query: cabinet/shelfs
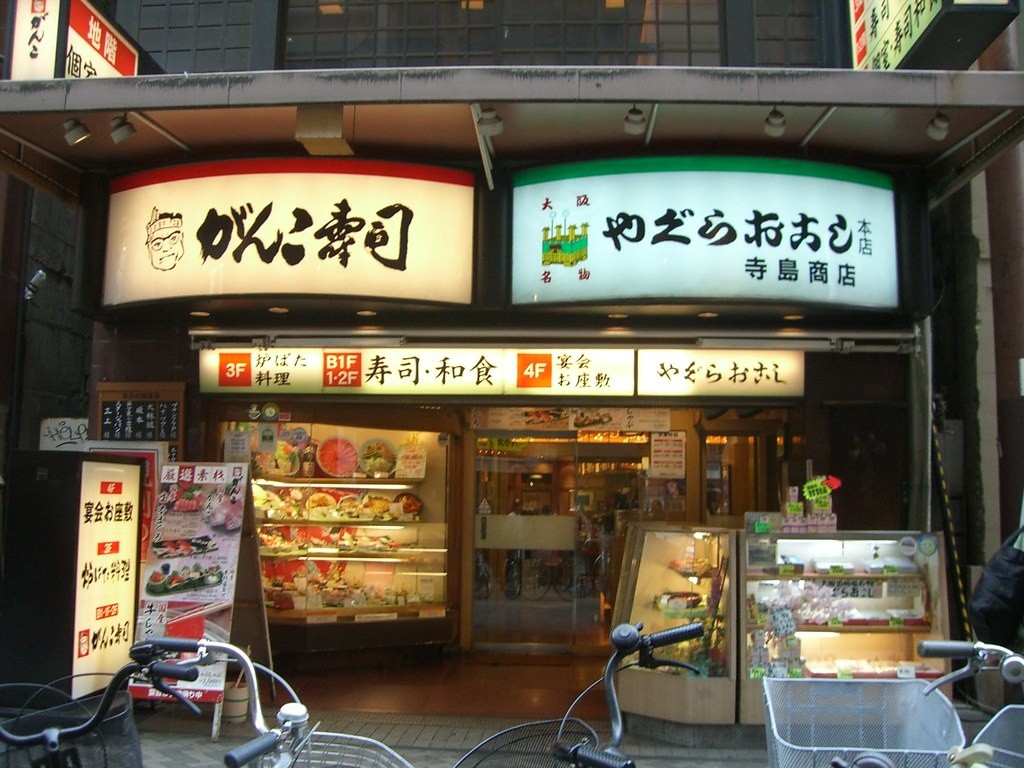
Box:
[609,524,738,727]
[737,529,954,726]
[202,400,464,654]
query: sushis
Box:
[160,538,193,557]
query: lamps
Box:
[476,101,505,137]
[924,108,951,142]
[622,104,647,135]
[110,112,136,144]
[62,119,92,147]
[762,105,787,138]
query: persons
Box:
[542,502,553,515]
[508,497,525,516]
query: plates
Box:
[357,437,398,478]
[305,492,424,522]
[316,435,360,478]
[145,571,223,597]
[285,452,300,476]
[151,535,219,557]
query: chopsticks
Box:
[165,602,231,625]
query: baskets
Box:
[762,676,966,768]
[256,731,416,768]
[453,717,599,768]
[971,704,1024,768]
[0,690,143,768]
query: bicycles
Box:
[0,642,203,768]
[761,639,1024,768]
[142,636,417,768]
[450,619,705,768]
[472,505,648,603]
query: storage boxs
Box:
[743,485,838,534]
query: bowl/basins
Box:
[136,607,229,643]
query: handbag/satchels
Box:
[967,525,1024,644]
